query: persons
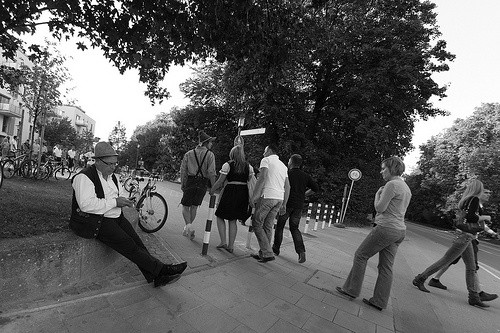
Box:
[240,166,254,225]
[412,178,500,308]
[209,146,256,252]
[70,142,188,288]
[271,154,319,263]
[2,135,163,179]
[250,145,290,263]
[180,130,217,239]
[335,157,412,310]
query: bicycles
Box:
[128,167,169,234]
[119,169,140,193]
[0,147,83,190]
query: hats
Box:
[196,134,217,148]
[91,142,119,158]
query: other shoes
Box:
[250,253,262,259]
[298,252,306,263]
[183,227,189,237]
[272,245,280,255]
[412,274,430,293]
[154,274,182,289]
[428,279,447,290]
[238,219,245,226]
[249,225,254,232]
[226,248,233,254]
[164,261,188,275]
[188,227,195,240]
[362,298,383,311]
[336,287,356,299]
[258,256,275,262]
[216,243,227,248]
[479,292,497,301]
[468,295,490,309]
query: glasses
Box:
[99,157,119,167]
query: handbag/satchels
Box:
[456,197,484,233]
[247,176,259,203]
